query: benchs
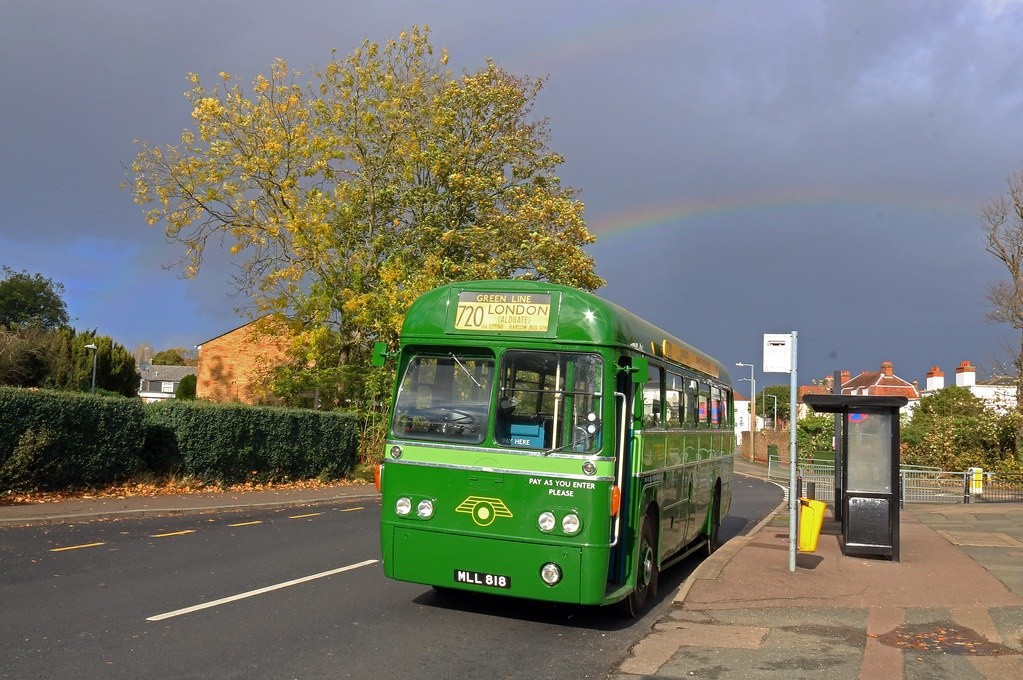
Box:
[516,411,728,429]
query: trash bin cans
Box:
[766,444,778,466]
[797,497,827,554]
[968,466,983,494]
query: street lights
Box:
[84,343,98,395]
[736,362,756,462]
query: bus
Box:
[371,280,737,620]
